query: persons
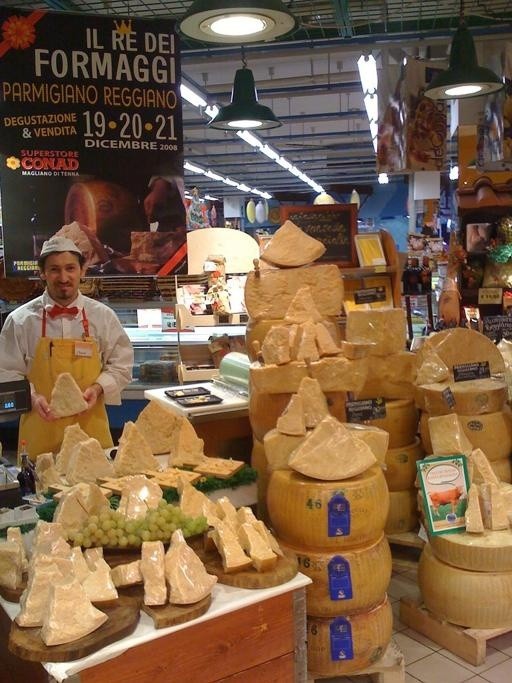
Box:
[0,234,135,462]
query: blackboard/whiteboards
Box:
[280,203,356,267]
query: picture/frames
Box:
[354,234,388,266]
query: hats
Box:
[40,237,82,257]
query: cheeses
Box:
[0,219,512,676]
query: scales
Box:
[0,380,32,412]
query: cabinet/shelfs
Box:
[255,227,402,348]
[402,257,433,340]
[438,124,512,345]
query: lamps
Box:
[356,48,389,185]
[180,71,325,202]
[207,46,282,132]
[423,0,504,101]
[177,0,300,43]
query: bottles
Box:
[17,455,36,497]
[423,314,469,335]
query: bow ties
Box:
[48,303,78,320]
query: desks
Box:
[144,379,252,458]
